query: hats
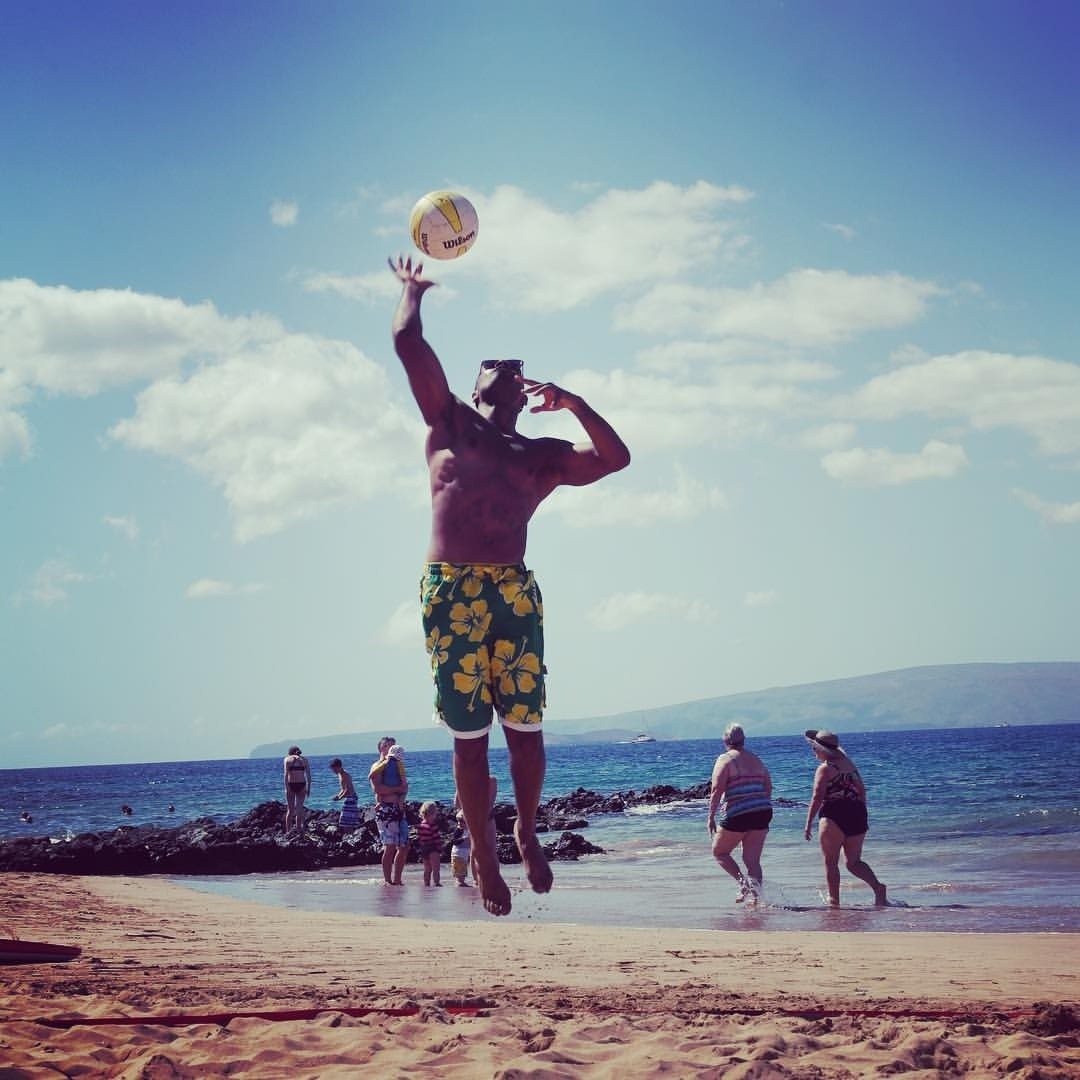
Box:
[387,745,405,761]
[804,728,847,758]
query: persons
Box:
[387,254,631,918]
[285,725,886,913]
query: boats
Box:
[630,731,655,745]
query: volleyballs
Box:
[409,188,479,260]
